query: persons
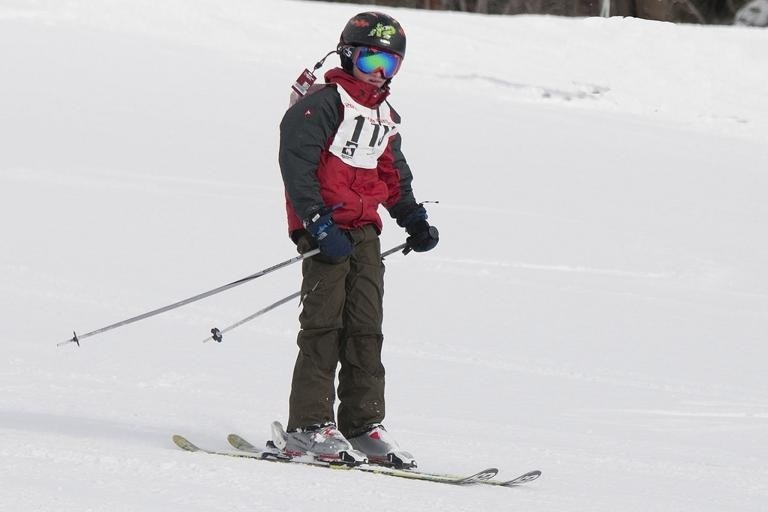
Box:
[276,12,440,467]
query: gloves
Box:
[305,213,354,257]
[405,217,439,253]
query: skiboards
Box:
[172,434,541,487]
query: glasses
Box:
[356,47,402,79]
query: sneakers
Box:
[348,423,418,469]
[286,422,367,463]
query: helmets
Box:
[338,11,406,58]
